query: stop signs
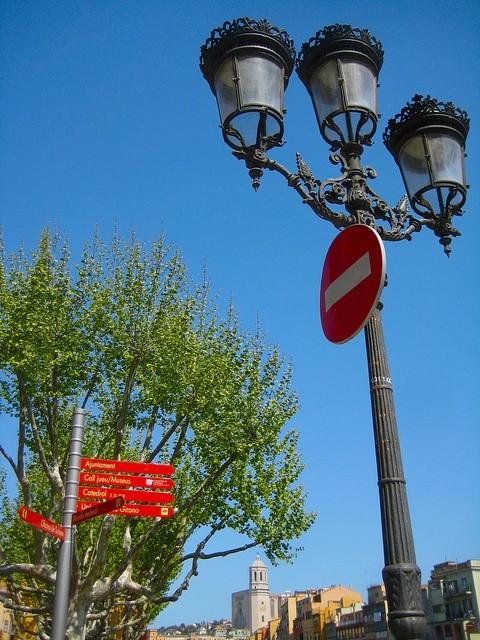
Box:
[317,223,387,345]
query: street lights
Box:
[197,8,473,636]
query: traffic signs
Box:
[78,485,173,503]
[81,472,173,490]
[72,496,122,522]
[77,501,172,518]
[19,508,64,541]
[81,458,174,475]
